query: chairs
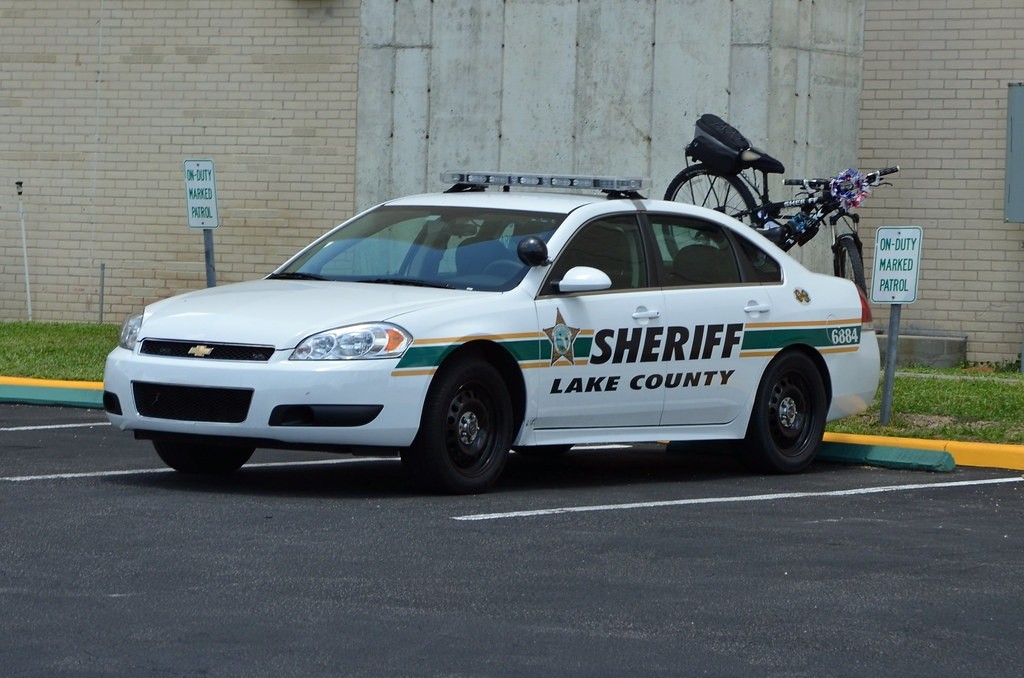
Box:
[454,237,507,277]
[672,244,727,287]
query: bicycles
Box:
[663,143,900,300]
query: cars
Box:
[102,171,880,489]
